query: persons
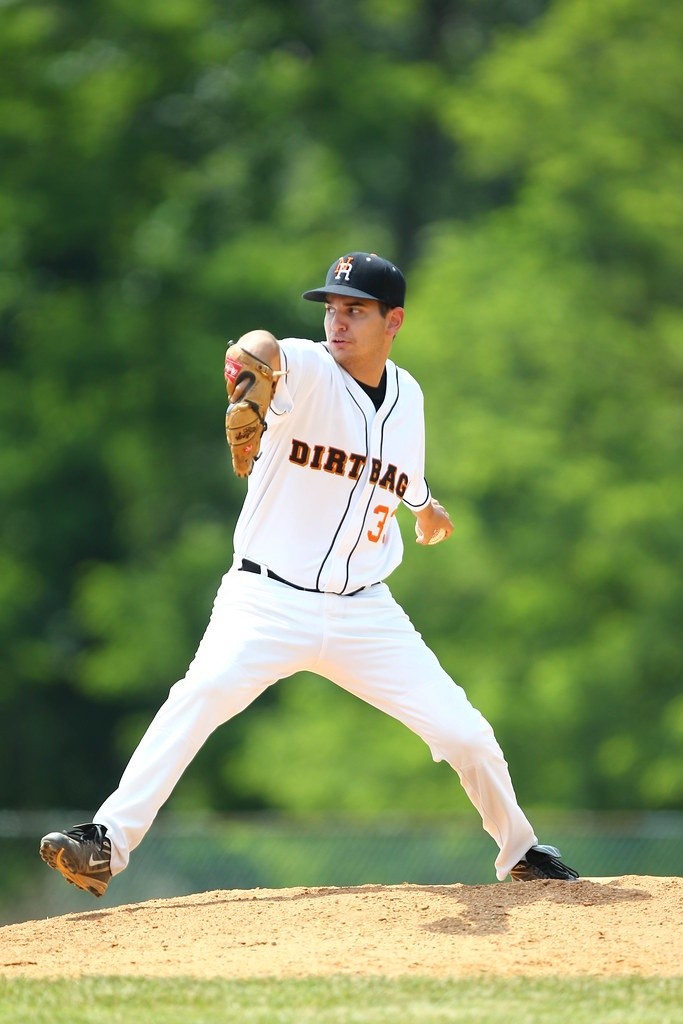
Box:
[40,251,579,897]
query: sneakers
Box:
[39,822,111,898]
[508,845,580,881]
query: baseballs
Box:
[414,518,447,546]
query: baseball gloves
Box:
[223,341,279,480]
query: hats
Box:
[302,252,407,309]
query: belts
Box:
[238,559,380,596]
